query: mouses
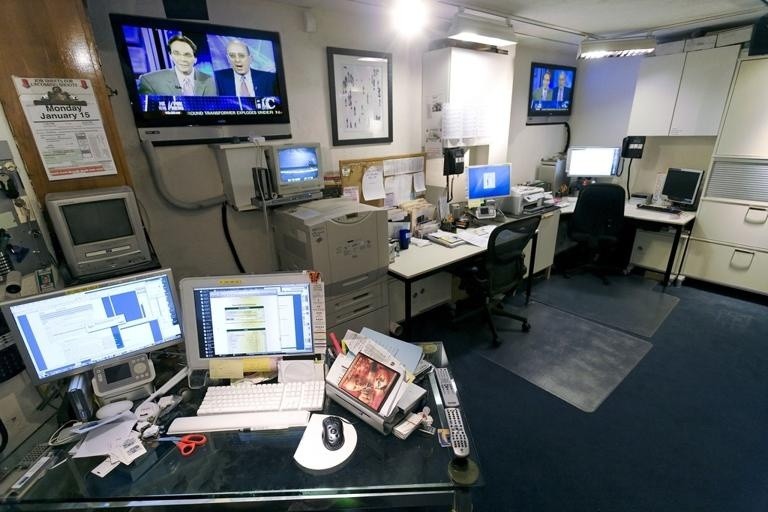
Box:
[321,416,345,451]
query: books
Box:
[325,326,449,440]
[399,198,465,248]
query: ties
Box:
[239,76,250,97]
[182,76,195,96]
[558,88,562,102]
[543,88,547,101]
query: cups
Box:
[399,229,411,250]
[441,220,456,234]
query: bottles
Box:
[554,179,587,199]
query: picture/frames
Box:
[326,46,394,146]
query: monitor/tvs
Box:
[179,272,326,370]
[46,186,151,279]
[525,62,577,127]
[465,162,512,201]
[565,145,621,178]
[265,143,326,196]
[108,12,294,142]
[661,168,705,209]
[0,267,183,388]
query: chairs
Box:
[566,183,627,286]
[457,215,542,348]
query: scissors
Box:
[154,433,207,457]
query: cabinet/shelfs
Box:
[388,273,452,324]
[625,43,741,137]
[680,53,767,296]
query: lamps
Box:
[446,12,519,49]
[575,38,656,62]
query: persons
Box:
[552,74,571,100]
[533,72,553,100]
[138,36,218,96]
[214,41,277,97]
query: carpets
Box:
[522,278,681,340]
[469,300,652,414]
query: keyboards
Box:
[637,202,682,214]
[197,380,326,418]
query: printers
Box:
[496,185,562,216]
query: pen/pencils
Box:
[443,213,454,223]
[327,332,347,358]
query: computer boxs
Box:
[539,159,570,198]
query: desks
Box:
[0,340,484,512]
[390,197,698,319]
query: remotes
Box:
[444,406,470,458]
[435,367,460,409]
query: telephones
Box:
[443,148,464,175]
[621,136,645,158]
